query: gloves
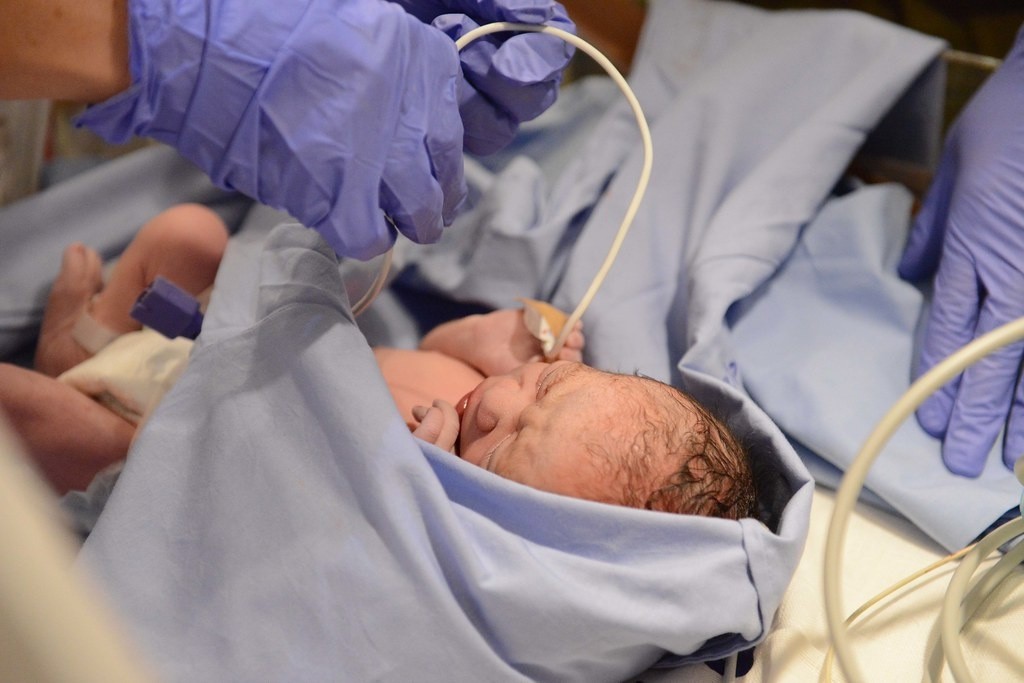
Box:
[897,26,1024,476]
[72,0,577,262]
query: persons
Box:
[0,203,759,521]
[2,4,576,261]
[896,28,1022,478]
